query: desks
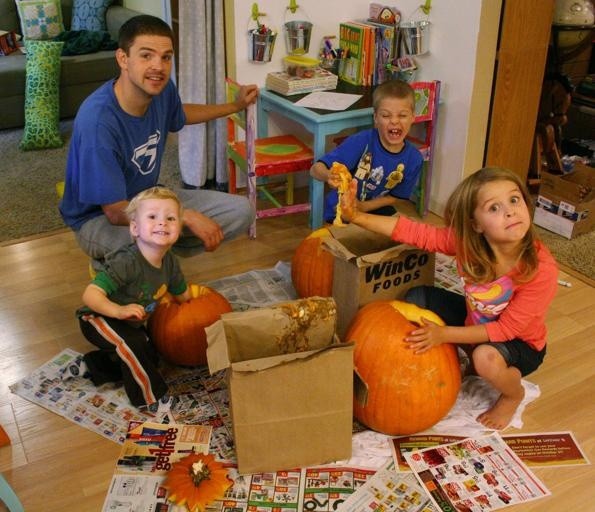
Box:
[256,85,384,234]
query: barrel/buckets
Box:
[245,12,278,64]
[400,5,431,57]
[282,6,313,55]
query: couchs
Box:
[0,0,148,130]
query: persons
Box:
[311,78,425,224]
[60,186,190,417]
[342,166,559,430]
[61,14,258,282]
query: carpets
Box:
[1,118,193,246]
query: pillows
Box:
[14,1,66,150]
[70,1,110,34]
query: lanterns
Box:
[292,224,341,298]
[345,300,463,436]
[149,284,233,364]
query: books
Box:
[265,18,396,97]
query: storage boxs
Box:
[534,161,595,239]
[321,219,438,341]
[205,293,361,473]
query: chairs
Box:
[406,81,440,219]
[222,80,314,238]
